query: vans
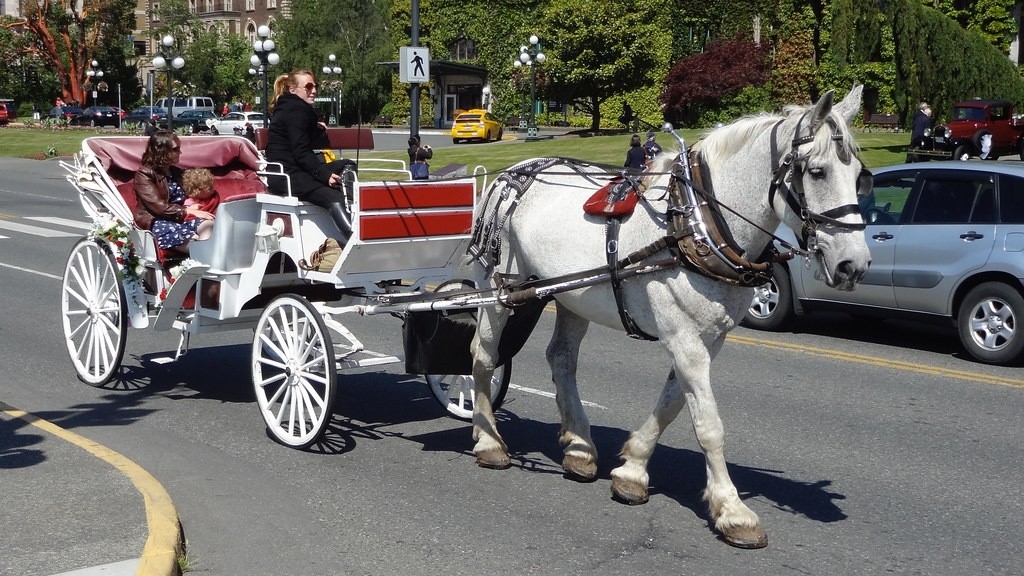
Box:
[155,97,214,119]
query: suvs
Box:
[124,106,173,131]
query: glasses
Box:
[173,147,180,152]
[296,83,317,91]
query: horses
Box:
[460,83,875,552]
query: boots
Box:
[337,164,358,197]
[326,199,353,236]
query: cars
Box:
[907,99,1024,161]
[744,161,1024,363]
[40,106,81,124]
[451,109,503,144]
[111,106,127,121]
[0,103,16,121]
[208,111,271,135]
[172,109,218,135]
[79,106,119,127]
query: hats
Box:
[645,132,656,140]
[245,122,253,126]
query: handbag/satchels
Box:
[410,147,429,179]
[299,238,341,273]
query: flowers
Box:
[89,223,186,318]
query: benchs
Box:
[862,115,899,133]
[116,178,269,264]
[255,125,412,183]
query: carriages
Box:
[59,82,873,552]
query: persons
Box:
[221,102,255,144]
[624,134,647,169]
[642,133,662,160]
[182,168,220,222]
[905,99,932,164]
[144,119,159,136]
[132,129,215,253]
[265,68,360,236]
[406,135,433,180]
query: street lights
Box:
[153,34,186,132]
[248,25,281,128]
[513,36,546,138]
[86,60,104,127]
[323,54,342,126]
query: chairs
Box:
[913,188,936,223]
